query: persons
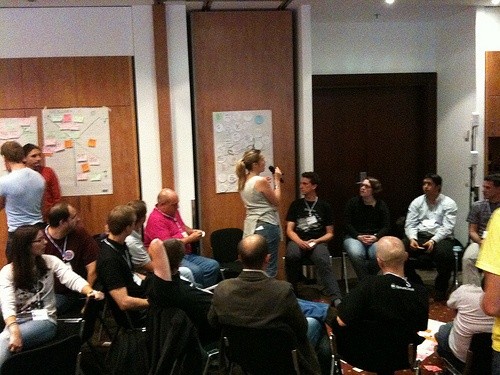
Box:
[285,172,344,307]
[207,235,338,375]
[331,236,429,347]
[343,177,391,281]
[0,141,49,264]
[237,149,285,279]
[434,173,500,375]
[0,143,61,223]
[0,187,225,369]
[400,173,457,301]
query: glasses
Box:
[32,235,46,243]
[360,183,371,188]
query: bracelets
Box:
[274,185,280,190]
[88,290,98,293]
[430,240,434,245]
[7,321,18,328]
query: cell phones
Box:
[309,241,316,247]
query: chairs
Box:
[0,228,491,375]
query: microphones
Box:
[269,166,284,183]
